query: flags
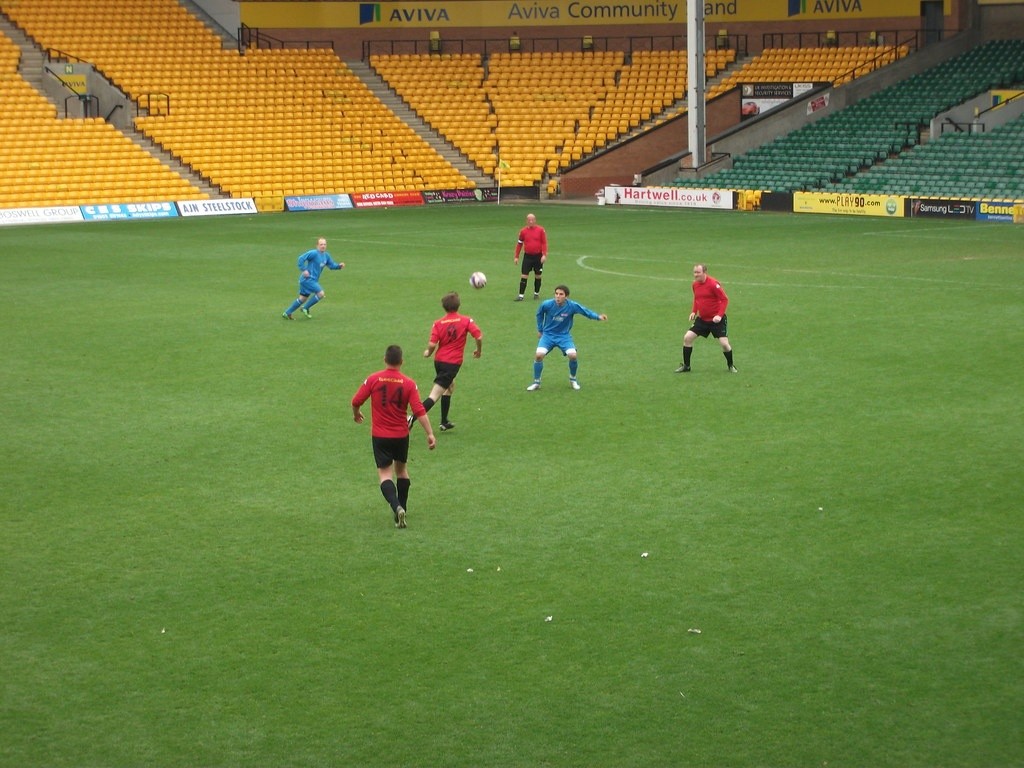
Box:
[501,160,510,167]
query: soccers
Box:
[470,272,487,289]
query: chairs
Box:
[0,1,1024,207]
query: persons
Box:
[351,345,436,528]
[408,292,482,431]
[514,214,546,301]
[282,238,344,321]
[675,264,737,373]
[527,285,607,390]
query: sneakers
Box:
[675,363,691,372]
[282,311,295,320]
[439,422,455,431]
[300,306,312,319]
[406,415,413,430]
[527,379,541,391]
[396,505,407,528]
[727,365,737,373]
[570,381,580,390]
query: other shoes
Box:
[514,296,524,301]
[534,294,539,299]
